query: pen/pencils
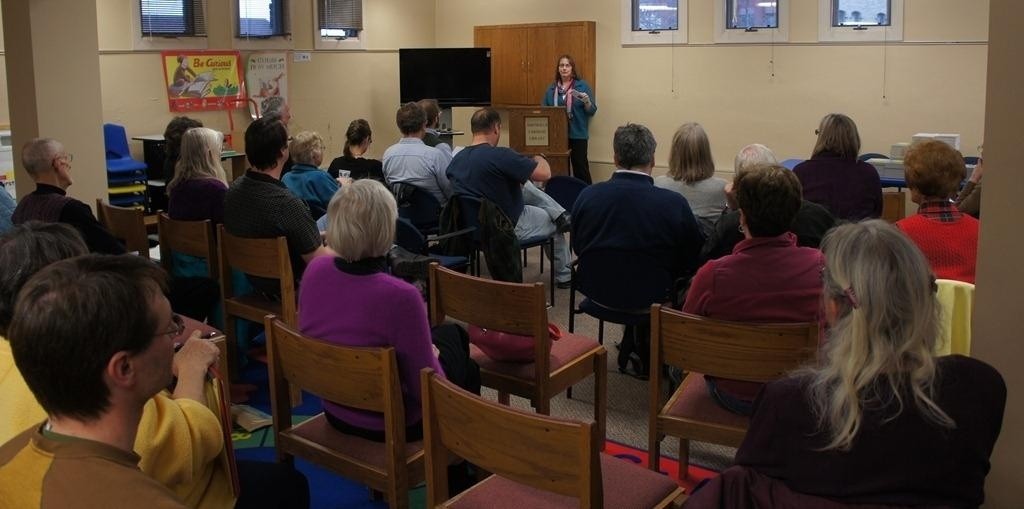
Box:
[174,331,216,353]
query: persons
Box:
[685,168,830,347]
[684,219,1008,509]
[954,138,985,218]
[539,53,595,182]
[654,121,730,224]
[172,56,195,84]
[795,111,883,226]
[1,138,310,509]
[701,143,834,264]
[569,124,703,381]
[167,97,576,289]
[300,179,481,498]
[893,140,979,284]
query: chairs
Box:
[643,301,821,473]
[262,314,457,508]
[169,311,243,500]
[427,263,612,450]
[214,223,302,385]
[441,192,557,308]
[157,209,218,332]
[100,122,150,200]
[92,200,157,264]
[564,257,648,398]
[383,181,440,253]
[413,364,683,507]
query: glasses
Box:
[52,153,73,165]
[152,313,184,338]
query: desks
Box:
[778,156,982,197]
[134,135,249,190]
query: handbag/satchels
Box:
[470,323,560,360]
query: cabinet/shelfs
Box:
[474,21,596,108]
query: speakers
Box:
[399,47,492,107]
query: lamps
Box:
[320,27,359,41]
